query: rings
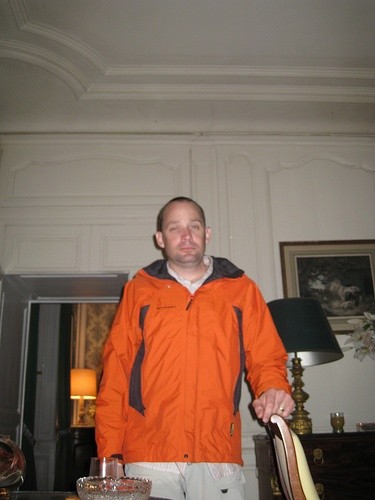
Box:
[278,407,283,412]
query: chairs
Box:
[269,414,321,500]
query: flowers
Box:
[343,312,375,362]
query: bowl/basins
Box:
[76,476,153,500]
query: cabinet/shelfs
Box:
[255,430,375,500]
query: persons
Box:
[95,197,295,500]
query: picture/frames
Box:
[279,239,375,333]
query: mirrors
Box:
[17,299,120,492]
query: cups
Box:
[330,413,344,433]
[89,456,125,477]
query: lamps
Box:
[266,297,344,434]
[70,368,97,425]
